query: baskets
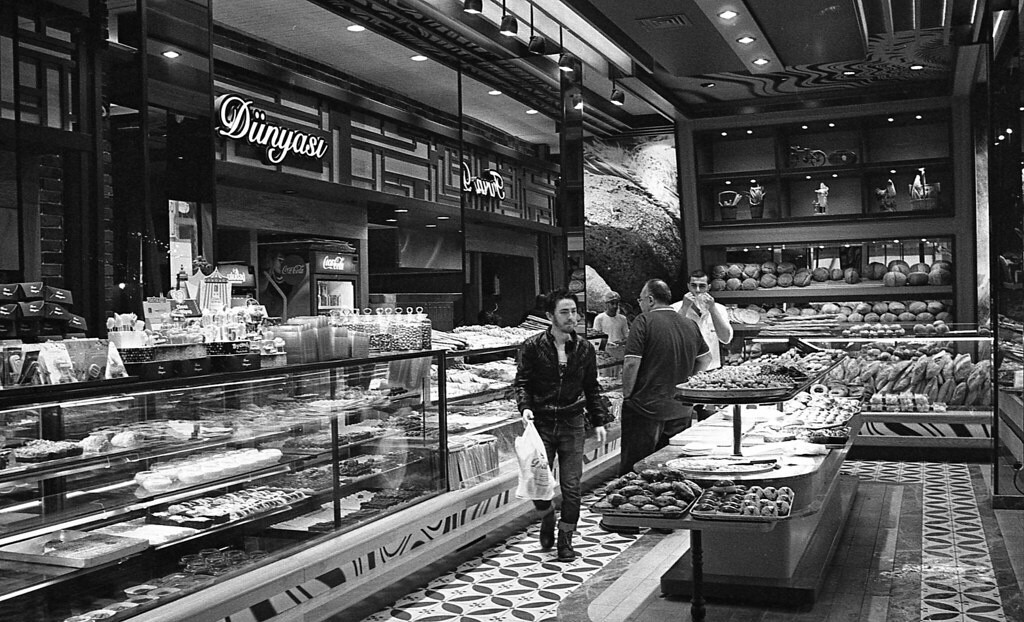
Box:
[156,343,212,359]
[211,341,250,355]
[117,341,157,362]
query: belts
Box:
[532,399,586,414]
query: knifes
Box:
[728,460,777,466]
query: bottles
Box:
[328,307,432,352]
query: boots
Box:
[540,510,556,548]
[557,530,575,562]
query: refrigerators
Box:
[214,262,255,307]
[259,251,361,318]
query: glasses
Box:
[637,296,649,303]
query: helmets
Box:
[605,291,621,304]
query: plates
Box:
[665,456,775,473]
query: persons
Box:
[514,290,607,562]
[477,270,734,423]
[599,278,712,535]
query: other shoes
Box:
[651,527,673,534]
[599,520,639,533]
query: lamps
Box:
[500,15,518,36]
[570,94,584,110]
[463,0,483,14]
[529,36,545,55]
[610,81,624,105]
[558,52,574,72]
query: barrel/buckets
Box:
[718,191,737,220]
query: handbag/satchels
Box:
[515,420,555,500]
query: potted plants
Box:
[719,191,742,221]
[746,185,766,219]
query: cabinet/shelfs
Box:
[675,99,976,366]
